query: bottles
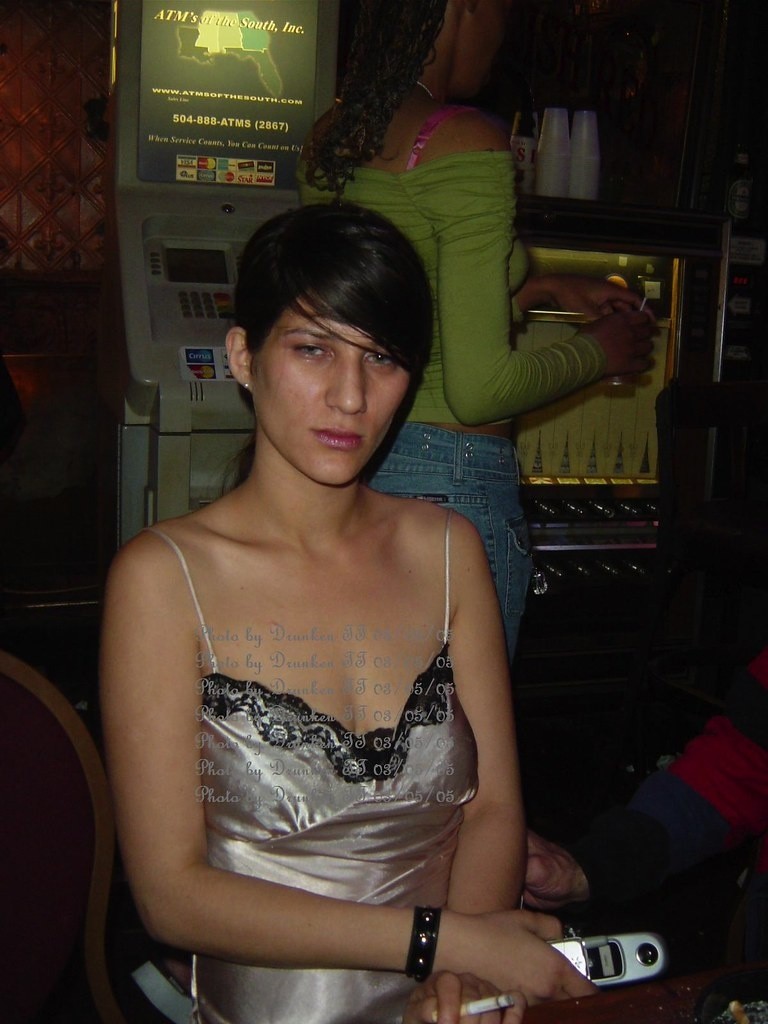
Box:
[720,126,754,234]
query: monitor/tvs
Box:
[137,0,320,191]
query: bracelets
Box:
[404,905,441,983]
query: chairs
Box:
[0,649,126,1024]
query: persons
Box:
[98,206,600,1023]
[520,641,768,1023]
[317,0,657,676]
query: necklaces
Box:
[414,77,436,100]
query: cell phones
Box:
[544,933,665,984]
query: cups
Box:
[602,375,635,386]
[510,111,597,201]
